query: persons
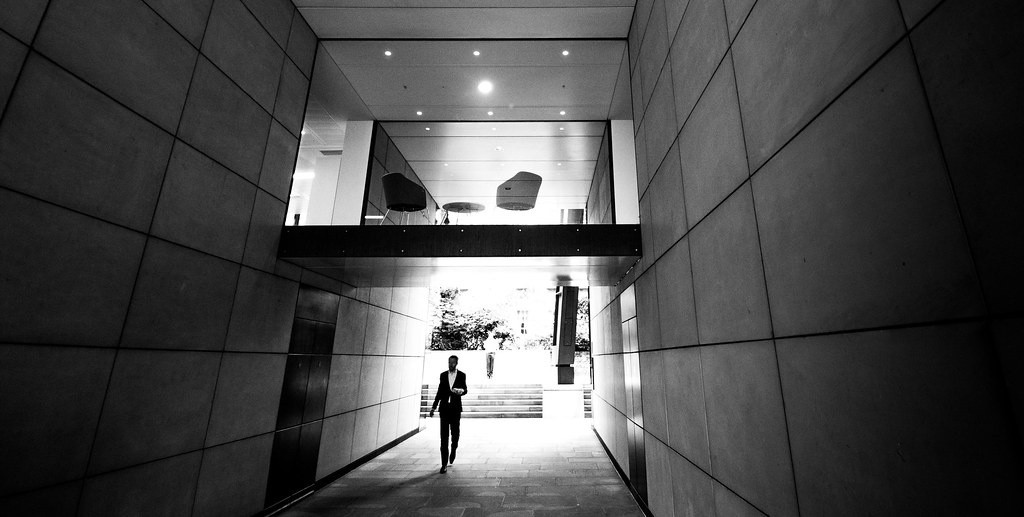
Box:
[483,333,499,379]
[430,355,468,474]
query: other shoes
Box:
[450,449,455,464]
[440,464,448,474]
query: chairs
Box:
[496,171,542,210]
[377,173,435,224]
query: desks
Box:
[438,201,485,224]
